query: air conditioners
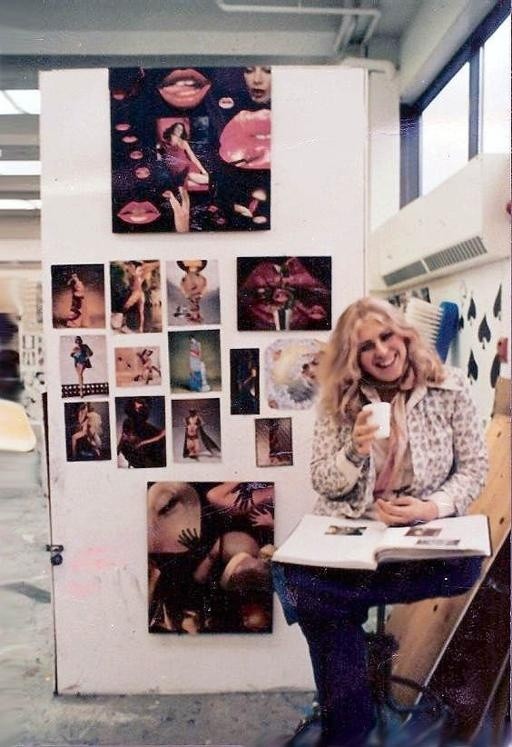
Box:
[371,153,512,293]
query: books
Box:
[271,513,494,571]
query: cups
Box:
[364,402,392,437]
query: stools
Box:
[290,575,478,746]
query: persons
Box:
[182,407,220,459]
[121,260,147,332]
[241,66,271,104]
[117,201,161,226]
[147,480,275,558]
[159,122,209,190]
[157,69,211,109]
[70,336,94,397]
[178,335,203,393]
[284,296,489,747]
